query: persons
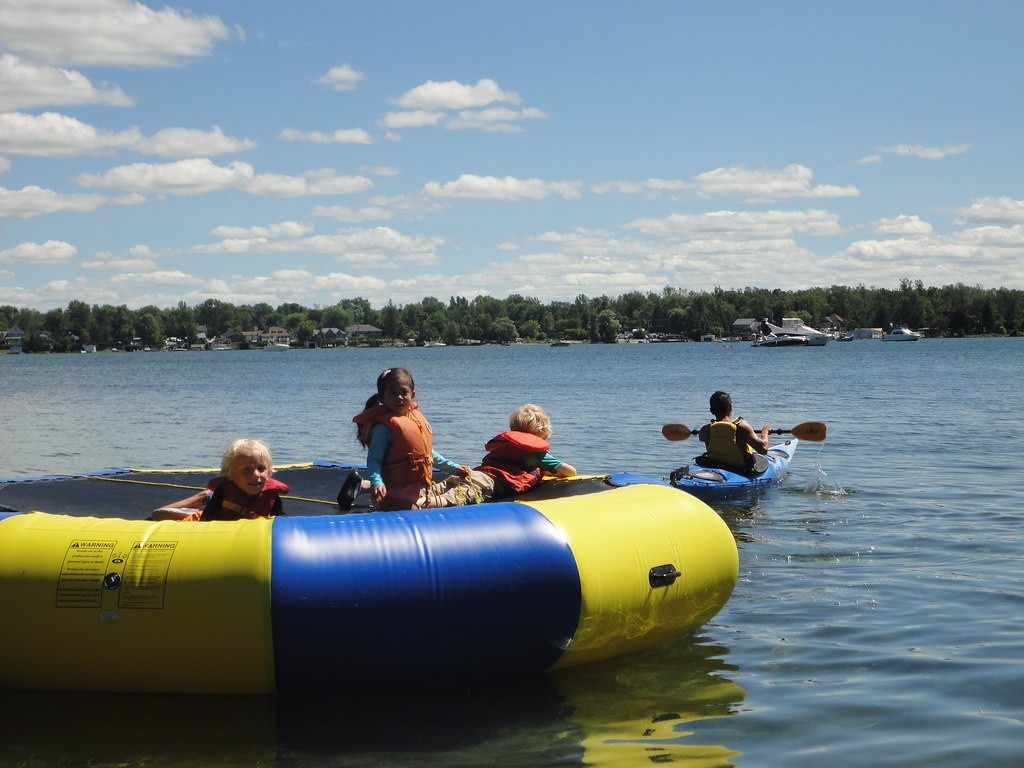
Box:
[338,404,576,510]
[700,391,772,470]
[151,438,289,521]
[352,368,472,501]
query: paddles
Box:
[661,421,828,442]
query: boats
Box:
[663,439,798,496]
[550,340,571,347]
[884,328,920,341]
[263,340,289,351]
[424,342,446,348]
[763,318,835,346]
[751,335,810,347]
[836,335,853,341]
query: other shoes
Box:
[337,470,362,510]
